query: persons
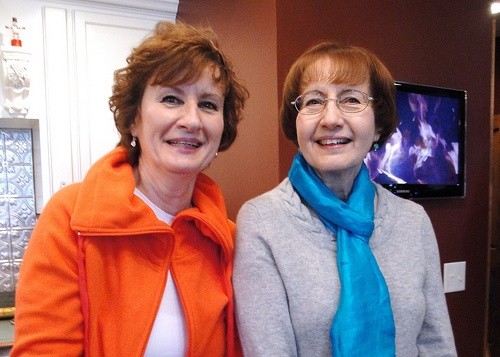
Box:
[231,41,458,357]
[8,19,250,357]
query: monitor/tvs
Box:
[363,81,467,199]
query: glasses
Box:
[291,92,376,114]
